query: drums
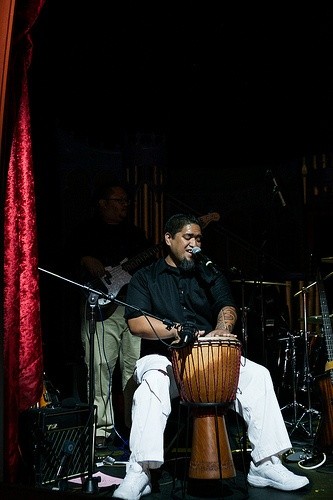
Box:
[239,304,266,343]
[274,334,306,382]
[170,336,241,479]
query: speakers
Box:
[19,404,98,485]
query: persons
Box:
[112,213,309,500]
[67,181,158,449]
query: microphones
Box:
[57,440,74,477]
[179,326,195,348]
[191,246,218,274]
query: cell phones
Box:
[280,281,321,438]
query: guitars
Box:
[88,211,221,305]
[315,274,333,448]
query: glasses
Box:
[106,197,131,204]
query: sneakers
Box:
[113,462,152,500]
[247,456,309,491]
